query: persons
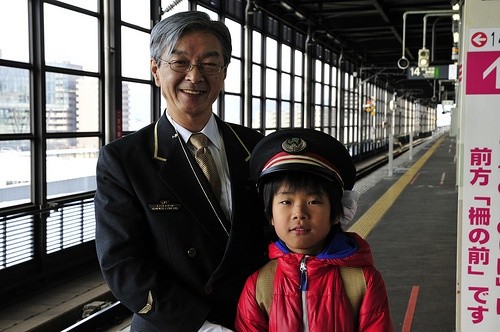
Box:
[234,127,395,332]
[96,10,359,332]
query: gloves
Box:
[338,189,360,228]
[199,320,233,332]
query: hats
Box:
[249,128,357,192]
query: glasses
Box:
[157,57,229,76]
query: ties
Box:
[189,133,222,204]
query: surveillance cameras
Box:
[398,58,409,69]
[432,97,437,102]
